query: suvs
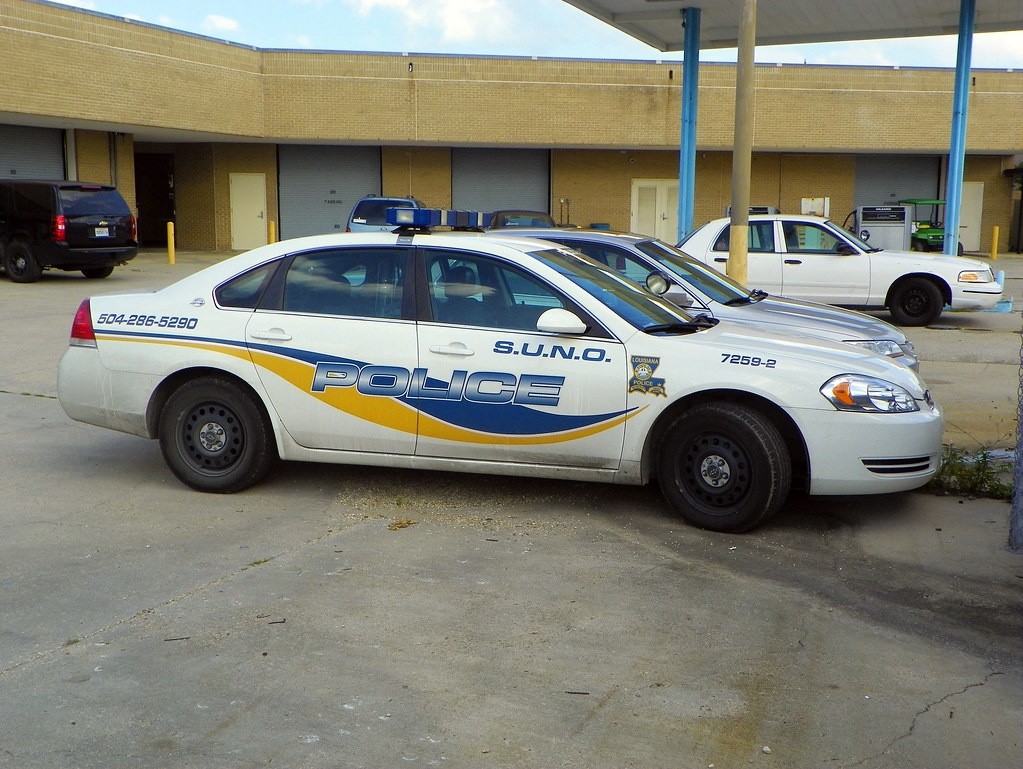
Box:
[345,194,428,259]
[0,178,139,283]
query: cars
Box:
[625,214,1002,327]
[483,210,559,231]
[53,207,941,534]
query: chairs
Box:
[441,266,482,325]
[311,275,355,316]
